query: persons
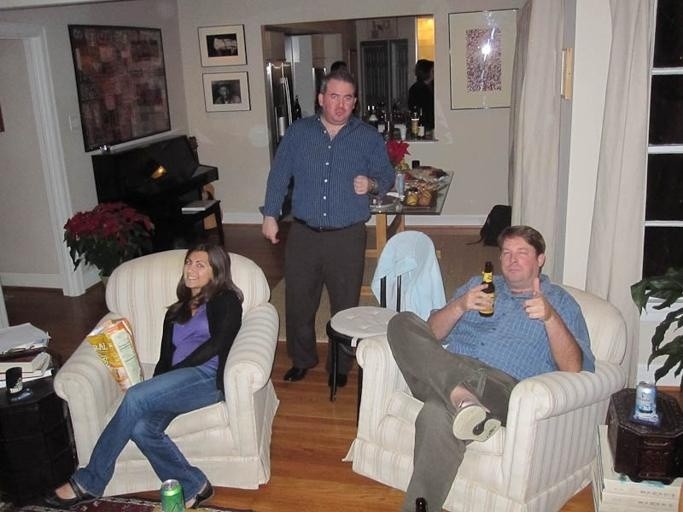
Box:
[41,242,242,509]
[387,225,595,511]
[258,70,394,387]
[330,61,347,71]
[215,83,240,103]
[409,59,435,135]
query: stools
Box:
[179,198,226,248]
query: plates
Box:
[612,388,683,439]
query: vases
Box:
[98,272,114,285]
[630,266,683,387]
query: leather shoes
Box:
[283,367,306,381]
[328,372,347,388]
[188,480,213,509]
[42,478,100,510]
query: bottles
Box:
[476,259,498,317]
[294,96,302,119]
[410,104,419,138]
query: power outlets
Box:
[69,113,81,135]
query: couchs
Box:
[341,282,628,511]
[53,249,280,500]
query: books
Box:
[180,200,218,211]
[0,352,54,389]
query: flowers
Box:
[63,201,156,276]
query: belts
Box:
[293,214,351,234]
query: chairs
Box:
[326,230,447,428]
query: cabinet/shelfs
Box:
[358,38,408,120]
[0,347,79,506]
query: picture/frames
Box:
[197,24,248,68]
[371,16,400,36]
[202,71,252,113]
[67,23,172,153]
[447,6,521,111]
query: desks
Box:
[359,168,454,306]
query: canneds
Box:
[633,381,659,416]
[158,479,185,512]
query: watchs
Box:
[370,178,378,195]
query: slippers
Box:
[450,403,503,442]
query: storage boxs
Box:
[605,389,683,485]
